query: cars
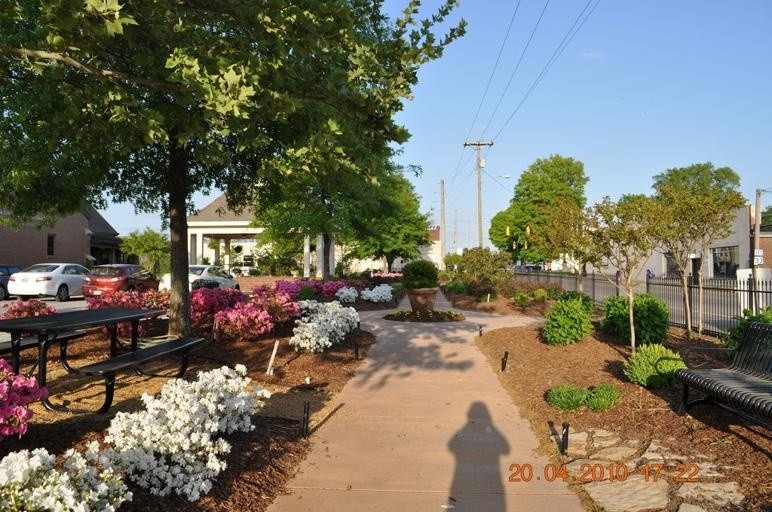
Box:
[0,263,240,301]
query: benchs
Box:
[654,318,772,451]
[75,337,206,413]
[2,330,89,384]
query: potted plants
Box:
[401,259,441,313]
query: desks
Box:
[0,306,166,414]
